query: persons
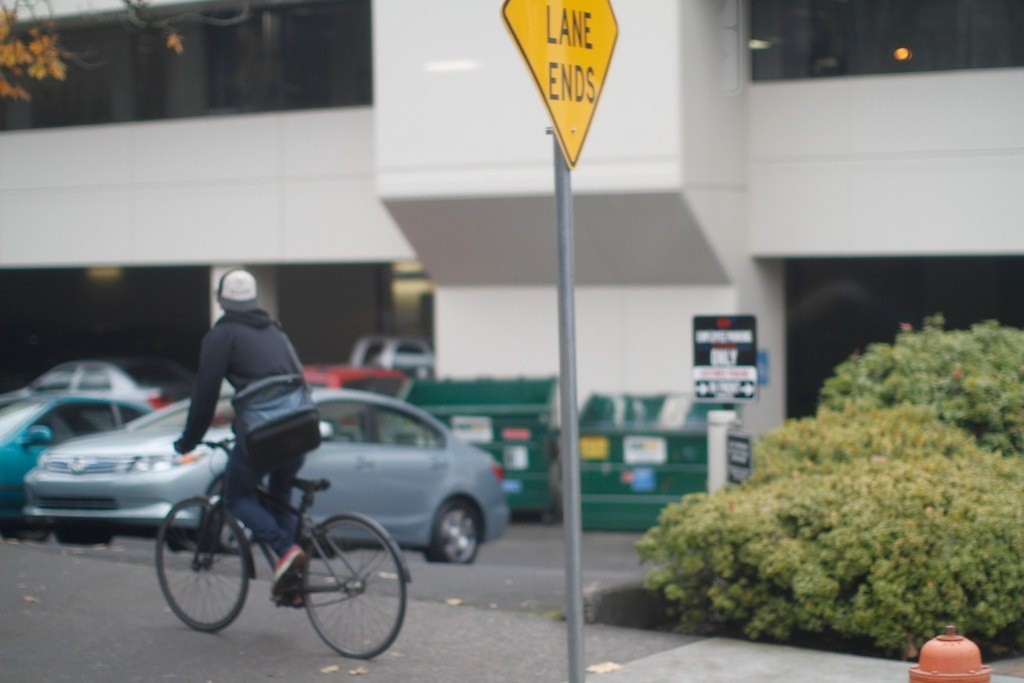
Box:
[172,269,323,610]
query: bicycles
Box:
[153,422,414,660]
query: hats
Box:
[216,268,257,313]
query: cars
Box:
[23,381,515,574]
[30,322,724,526]
[0,383,154,527]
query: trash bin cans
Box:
[394,367,561,524]
[566,387,741,534]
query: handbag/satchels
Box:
[232,374,322,466]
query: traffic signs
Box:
[727,429,754,491]
[692,313,760,404]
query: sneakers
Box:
[271,546,306,608]
